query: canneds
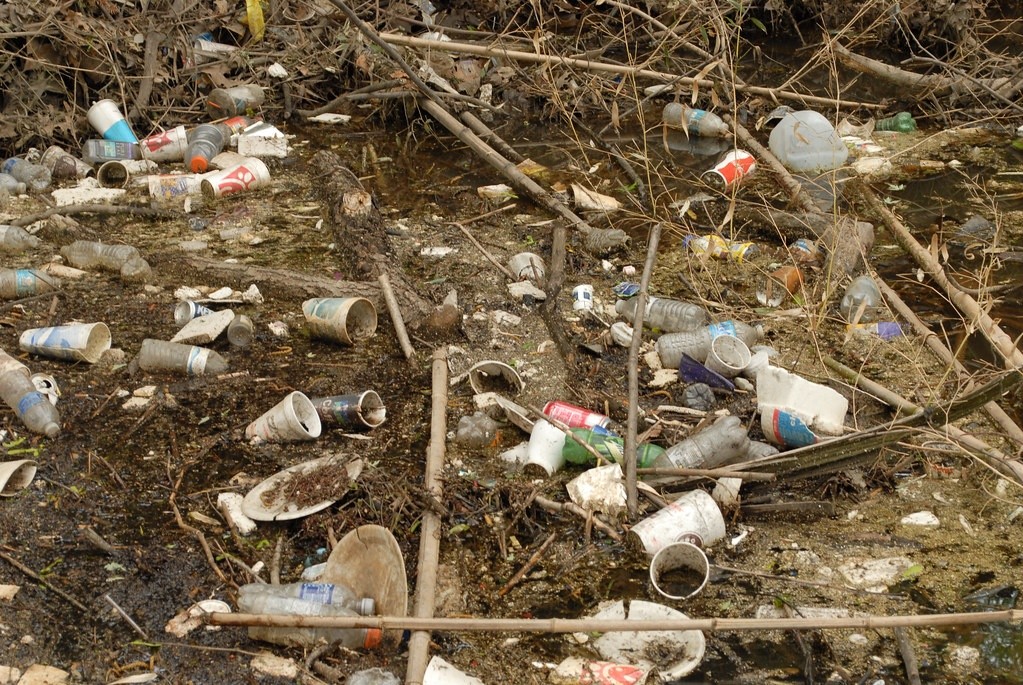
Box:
[545,401,612,431]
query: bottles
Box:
[0,39,919,650]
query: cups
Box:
[552,656,663,685]
[0,23,932,597]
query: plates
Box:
[243,453,363,524]
[576,600,706,680]
[322,526,407,648]
